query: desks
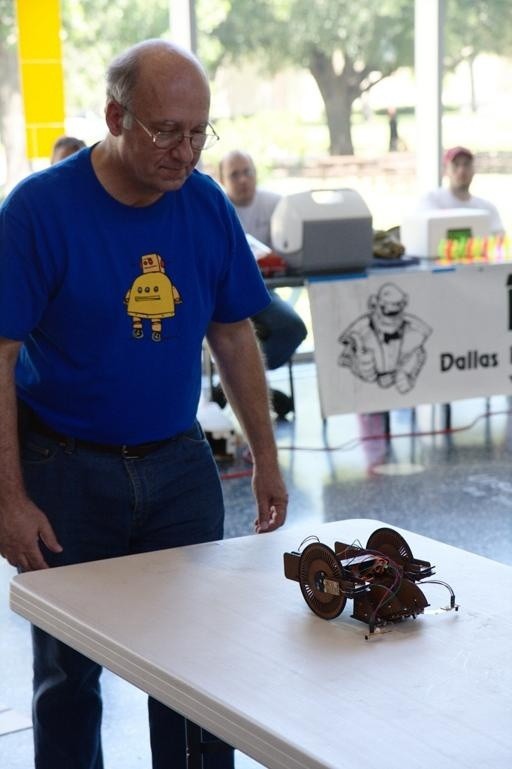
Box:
[195,252,511,464]
[7,514,512,769]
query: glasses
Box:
[123,106,221,151]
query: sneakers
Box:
[267,387,292,417]
[210,382,227,410]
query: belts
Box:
[23,404,196,461]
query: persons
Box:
[48,134,87,165]
[0,35,290,768]
[422,144,504,241]
[213,146,308,419]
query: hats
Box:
[443,145,473,166]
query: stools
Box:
[204,332,299,416]
